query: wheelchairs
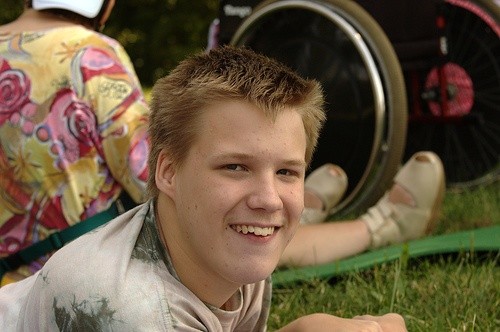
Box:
[205,1,500,220]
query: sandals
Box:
[362,150,447,251]
[296,162,348,225]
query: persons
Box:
[0,46,409,332]
[0,0,446,271]
